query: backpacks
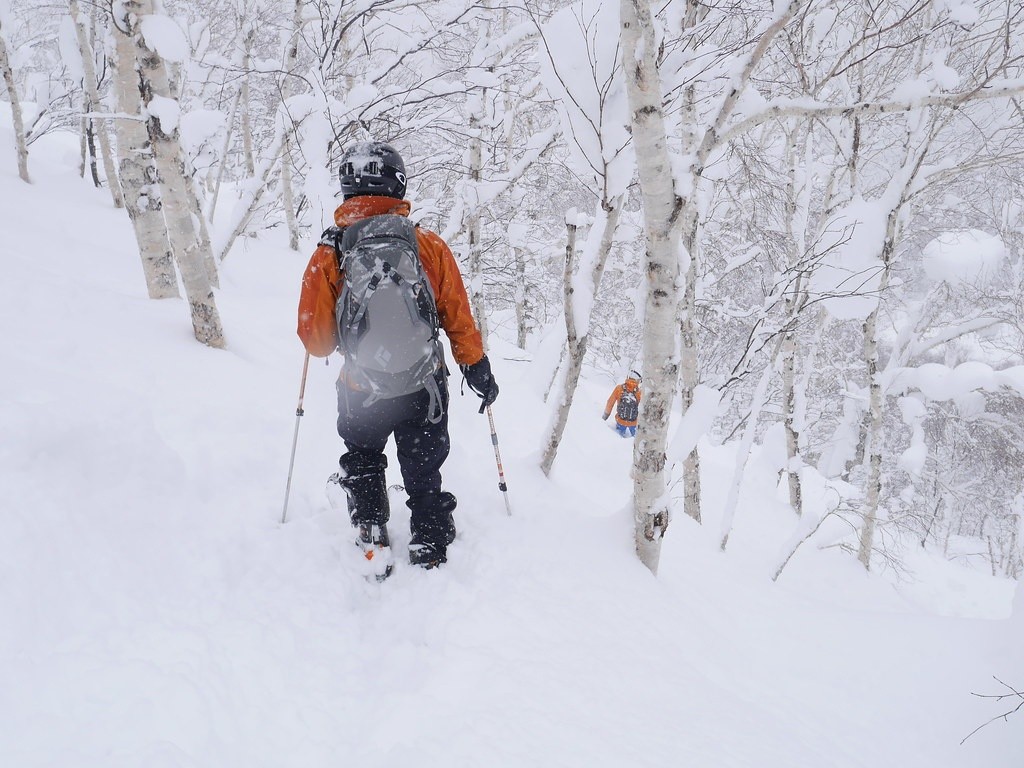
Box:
[316,213,450,423]
[616,383,640,423]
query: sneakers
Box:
[338,470,392,582]
[405,492,456,570]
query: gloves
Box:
[459,352,499,414]
[602,412,608,421]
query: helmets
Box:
[338,142,407,201]
[628,367,643,382]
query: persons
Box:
[602,370,641,464]
[297,142,498,584]
